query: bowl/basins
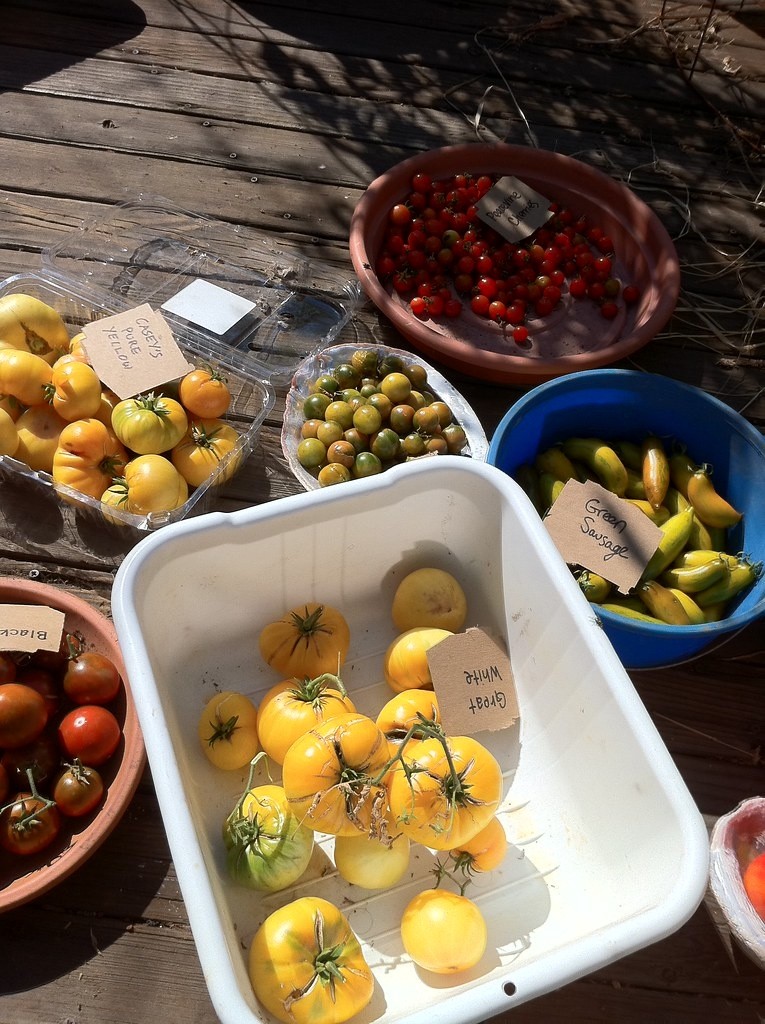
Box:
[281,343,487,492]
[0,190,376,531]
[112,455,712,1024]
[711,797,765,975]
[484,367,764,671]
[1,574,146,916]
[349,141,679,383]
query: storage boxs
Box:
[106,452,716,1023]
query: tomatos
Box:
[0,166,639,1024]
